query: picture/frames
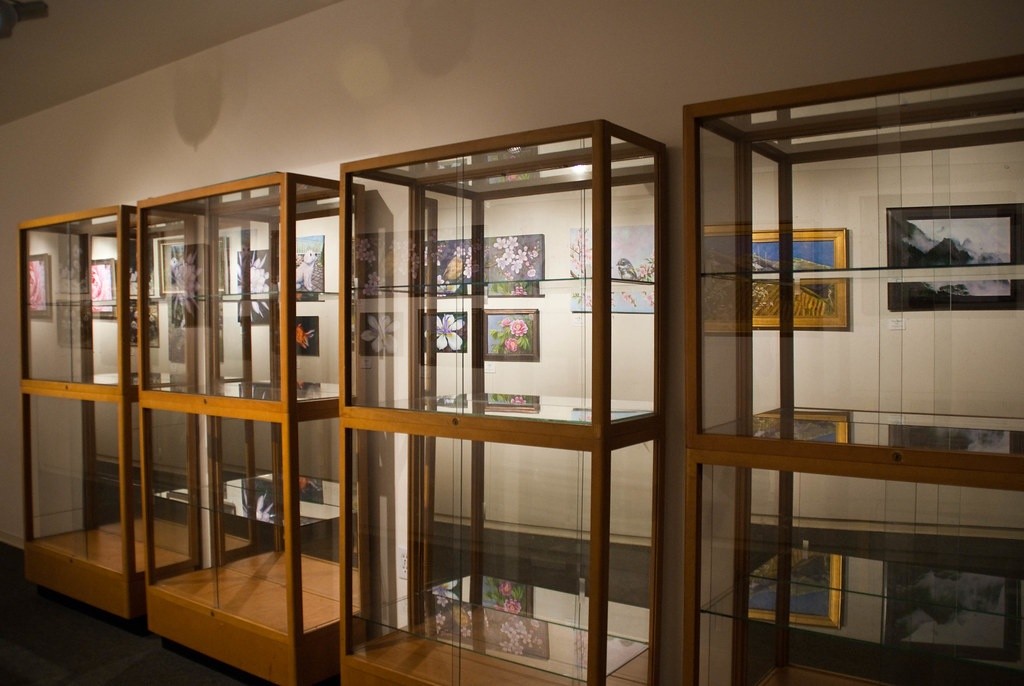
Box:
[92,259,118,319]
[28,253,53,319]
[299,477,324,505]
[752,228,849,330]
[431,594,474,647]
[483,606,550,661]
[481,308,540,363]
[296,315,321,357]
[482,576,533,620]
[436,313,466,354]
[240,476,277,524]
[746,411,850,631]
[886,202,1023,312]
[432,578,463,602]
[485,234,546,296]
[882,424,1019,662]
[158,240,231,295]
